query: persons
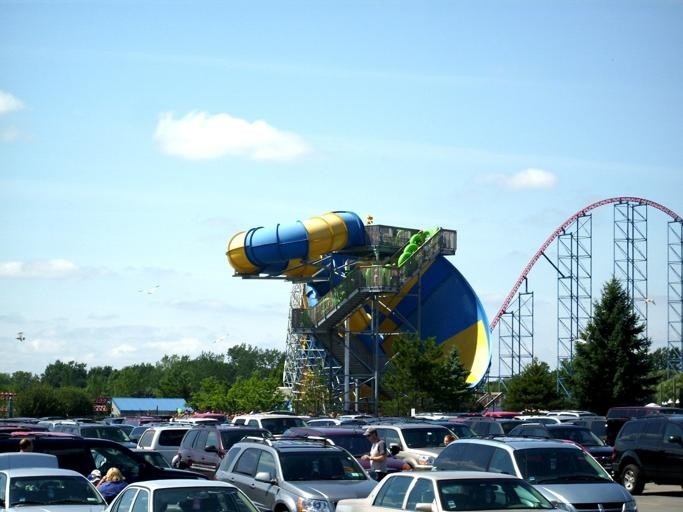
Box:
[363,429,386,480]
[97,468,127,499]
[443,437,452,446]
[20,439,34,452]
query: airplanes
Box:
[15,331,27,343]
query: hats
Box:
[91,469,102,477]
[362,427,377,436]
